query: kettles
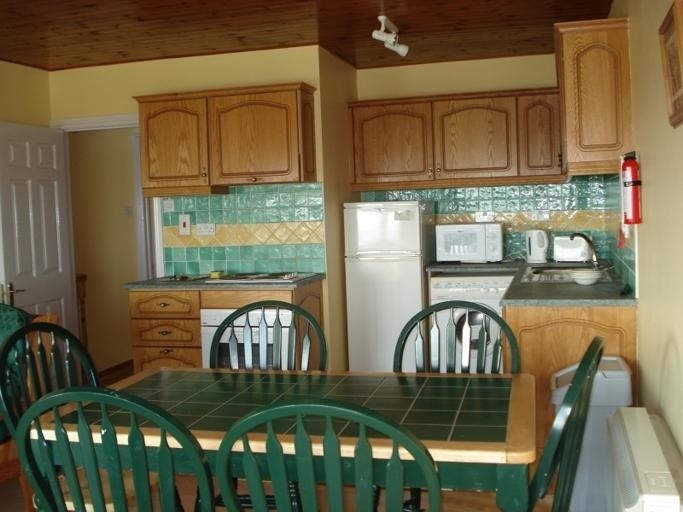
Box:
[524,229,548,264]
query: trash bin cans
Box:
[549,355,634,512]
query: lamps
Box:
[371,15,414,59]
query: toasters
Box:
[550,232,593,262]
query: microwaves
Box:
[434,223,503,263]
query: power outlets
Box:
[196,222,216,236]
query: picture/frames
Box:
[656,0,681,127]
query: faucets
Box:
[570,233,598,266]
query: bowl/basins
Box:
[570,268,601,285]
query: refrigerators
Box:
[340,199,435,374]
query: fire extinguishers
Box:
[621,150,641,224]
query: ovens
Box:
[200,308,293,371]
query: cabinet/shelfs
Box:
[126,290,200,376]
[499,302,636,497]
[551,17,633,176]
[517,87,569,183]
[74,270,90,386]
[349,89,517,189]
[131,80,316,200]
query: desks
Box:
[27,367,535,511]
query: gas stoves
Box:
[206,271,317,282]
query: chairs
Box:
[216,403,443,511]
[196,298,325,512]
[13,386,213,511]
[441,337,603,512]
[391,300,518,510]
[0,321,184,512]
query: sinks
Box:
[520,266,614,283]
